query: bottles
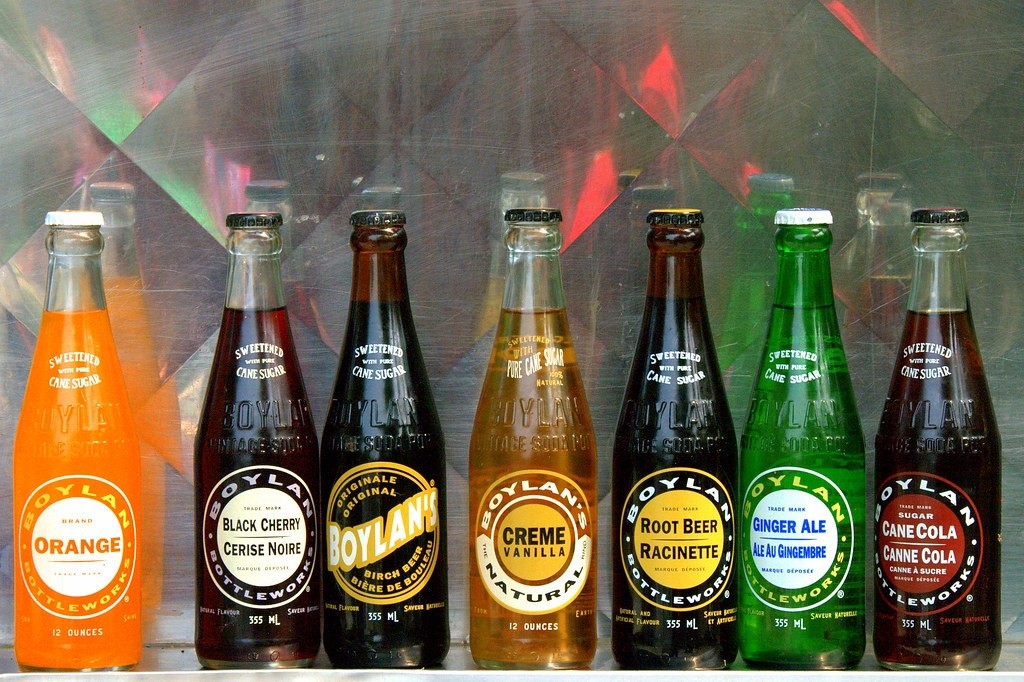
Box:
[14,210,142,671]
[322,210,450,668]
[92,182,164,620]
[483,172,549,340]
[874,209,1002,671]
[845,172,917,356]
[630,185,678,302]
[611,209,736,670]
[361,184,406,215]
[737,208,867,670]
[193,213,319,670]
[719,172,796,436]
[469,208,598,670]
[243,180,316,340]
